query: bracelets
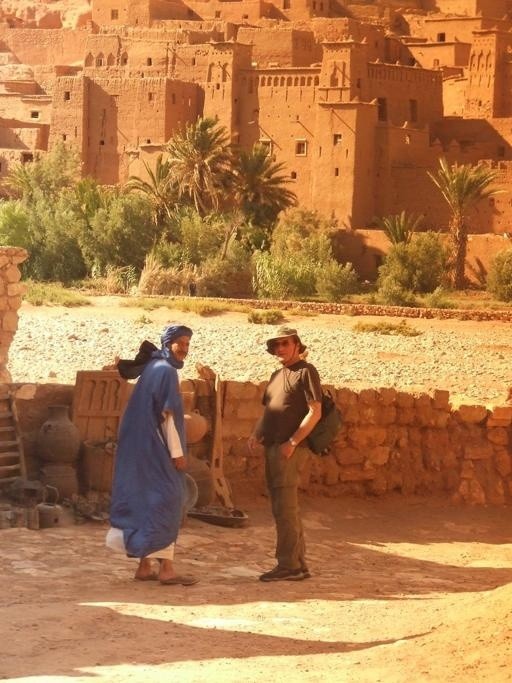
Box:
[289,436,299,450]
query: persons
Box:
[110,323,199,586]
[188,278,197,296]
[246,324,323,580]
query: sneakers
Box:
[259,565,310,582]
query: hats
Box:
[268,326,306,355]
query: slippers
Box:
[135,570,159,579]
[161,574,200,585]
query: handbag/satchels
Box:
[301,390,344,455]
